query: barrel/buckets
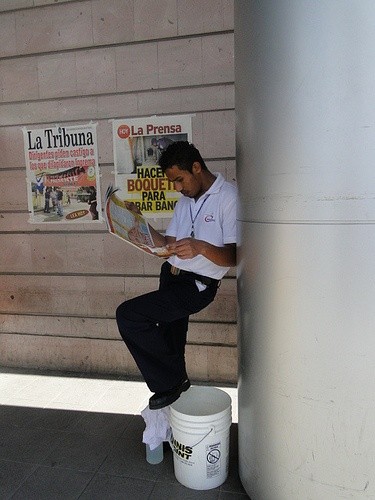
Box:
[171,385,232,490]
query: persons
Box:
[115,139,244,447]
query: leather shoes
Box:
[146,376,192,411]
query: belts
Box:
[166,265,218,288]
